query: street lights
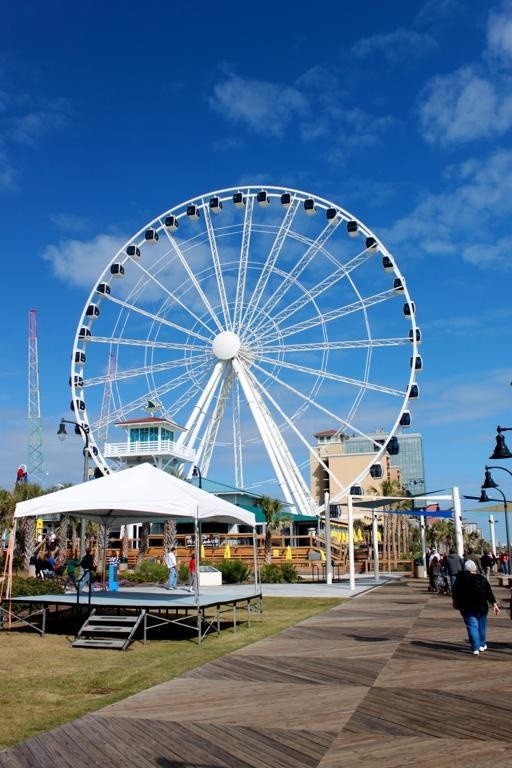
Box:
[477,422,511,577]
[55,417,92,578]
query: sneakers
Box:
[473,643,487,655]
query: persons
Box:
[30,547,99,594]
[426,545,511,656]
[110,549,120,580]
[188,553,196,593]
[187,537,218,546]
[165,547,181,590]
